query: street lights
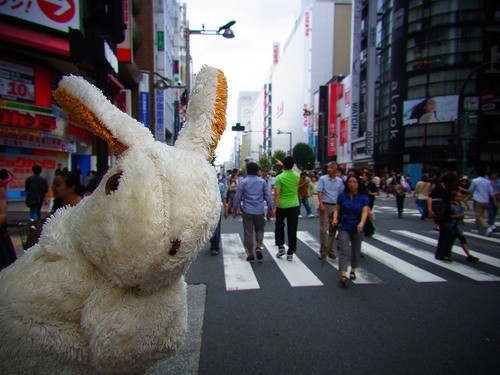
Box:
[154,27,235,113]
[277,131,292,156]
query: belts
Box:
[322,202,336,206]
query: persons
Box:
[0,162,108,270]
[409,98,439,123]
[210,156,500,283]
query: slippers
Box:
[350,272,356,279]
[340,275,347,284]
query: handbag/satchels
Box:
[401,175,410,191]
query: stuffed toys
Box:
[0,66,228,375]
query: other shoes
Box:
[246,255,254,260]
[276,247,285,257]
[433,226,439,230]
[287,254,293,261]
[471,230,479,233]
[437,256,452,262]
[399,214,403,216]
[486,228,493,237]
[328,249,335,259]
[467,255,479,261]
[212,250,219,255]
[491,224,496,229]
[421,212,426,220]
[255,247,264,260]
[360,253,364,258]
[264,213,315,222]
[318,253,327,260]
